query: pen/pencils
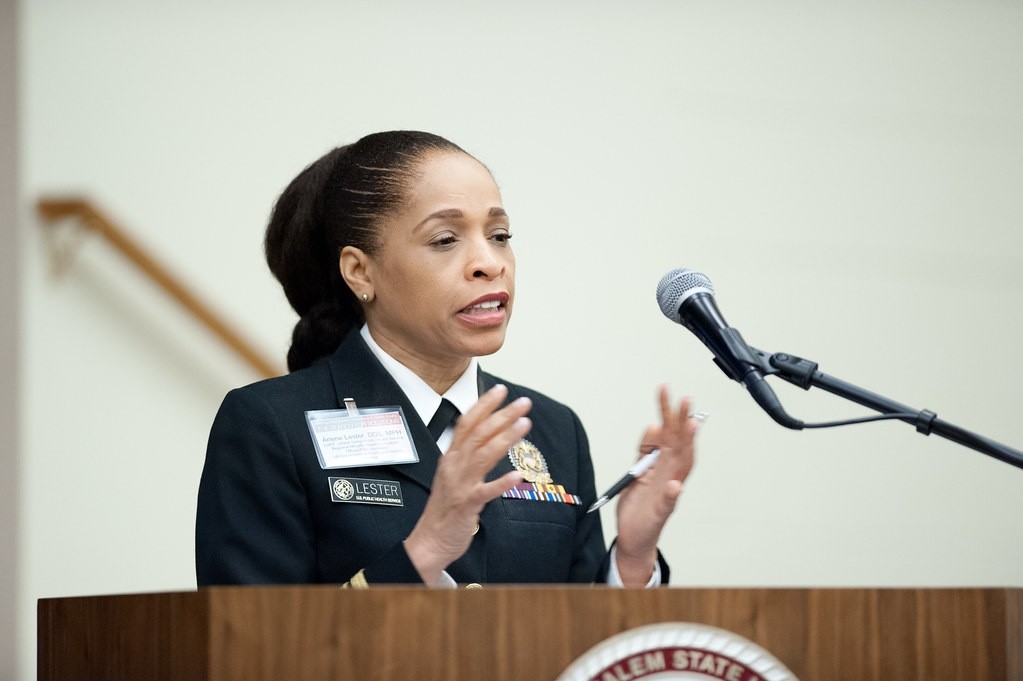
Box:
[586,413,707,513]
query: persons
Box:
[195,129,696,589]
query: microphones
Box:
[656,266,804,430]
